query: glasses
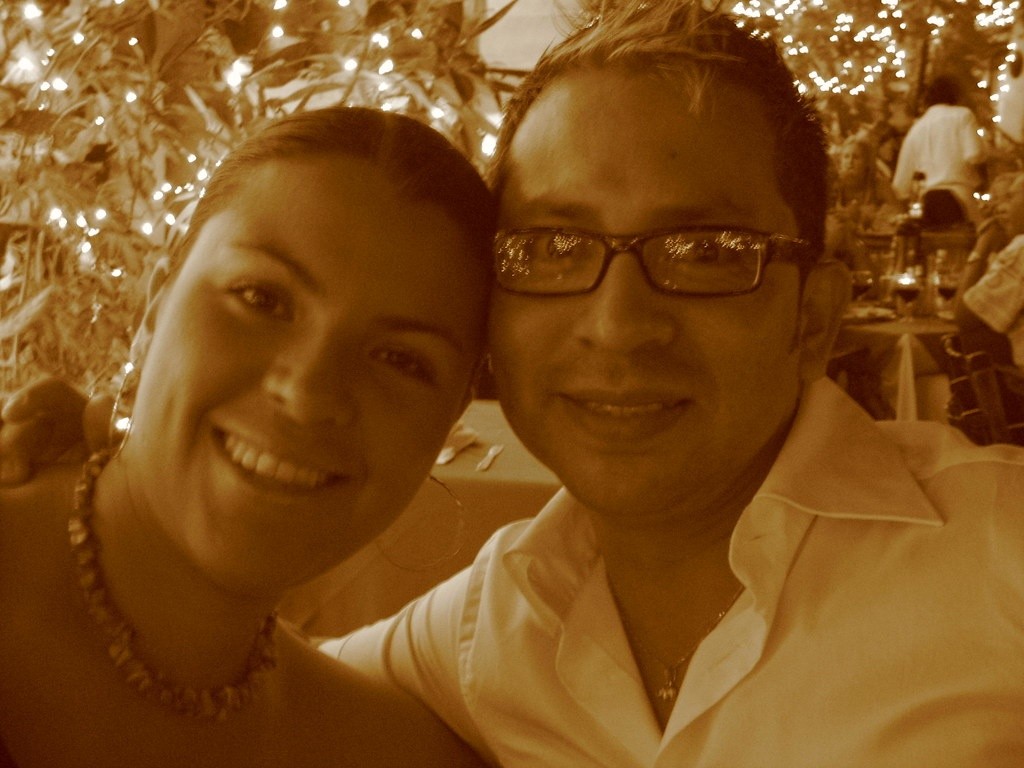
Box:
[479,224,819,301]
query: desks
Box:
[841,321,1007,446]
[277,396,561,639]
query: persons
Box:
[1,1,1023,766]
[0,106,489,767]
[892,72,1024,444]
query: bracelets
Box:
[969,248,981,267]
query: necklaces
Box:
[627,583,746,697]
[69,447,279,719]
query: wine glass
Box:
[939,275,957,319]
[898,274,920,324]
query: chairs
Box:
[939,334,1011,445]
